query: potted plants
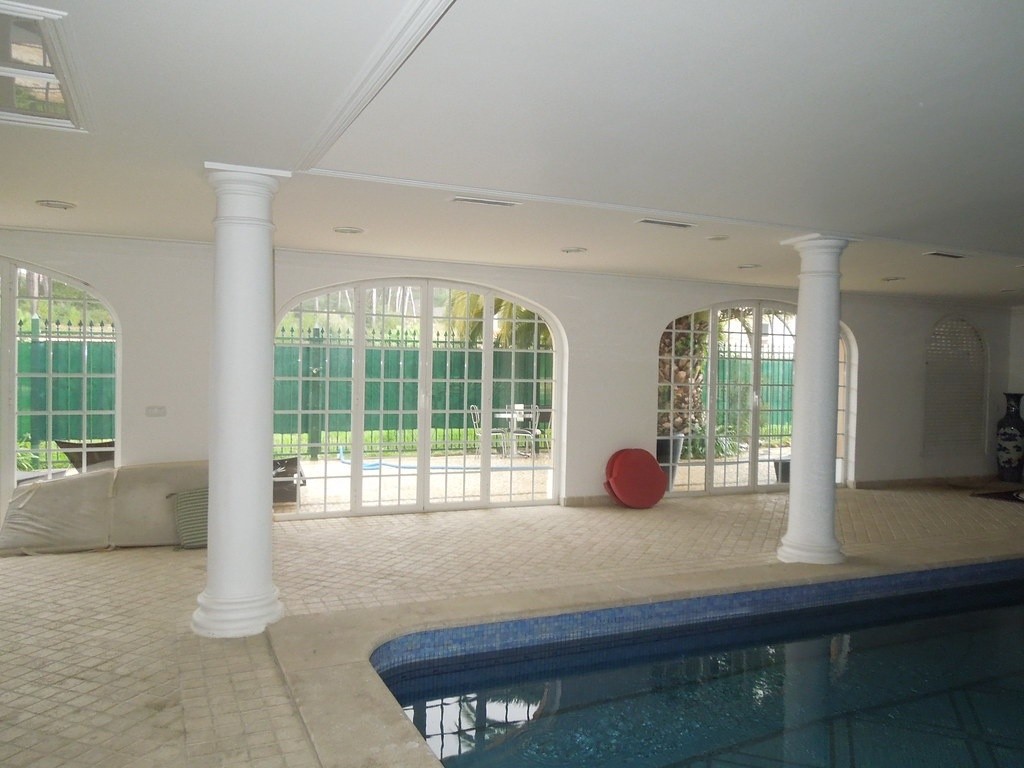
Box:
[656,308,724,491]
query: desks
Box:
[494,411,533,459]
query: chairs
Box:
[468,400,551,460]
